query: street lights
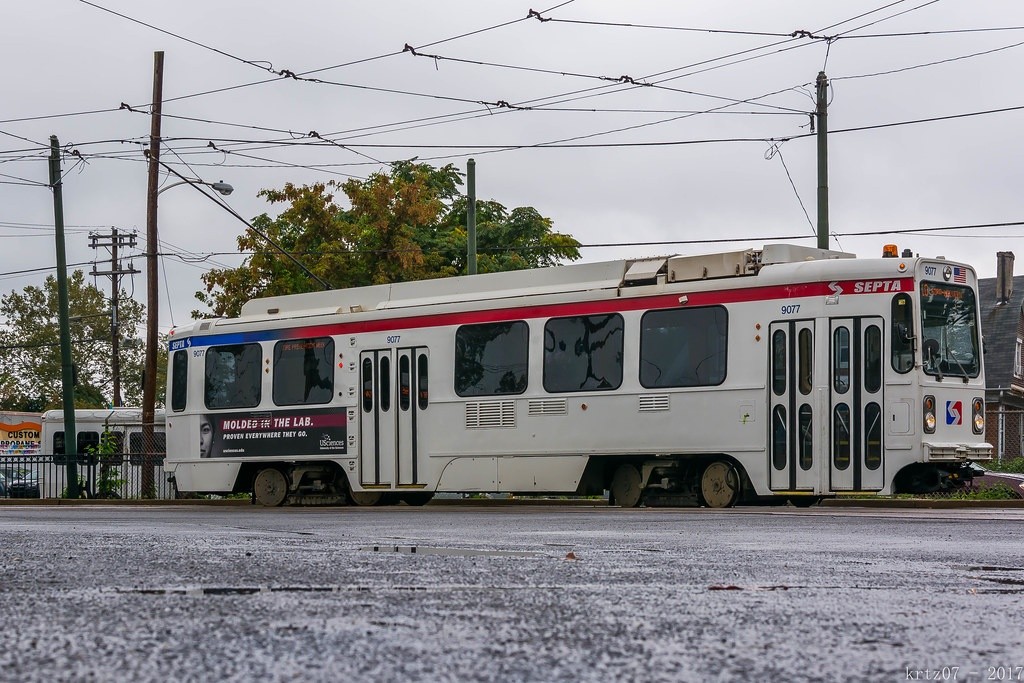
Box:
[141,180,234,496]
[69,311,121,408]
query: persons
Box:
[200,414,216,458]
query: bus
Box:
[40,409,221,500]
[160,243,996,506]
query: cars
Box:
[0,467,24,497]
[7,470,39,498]
